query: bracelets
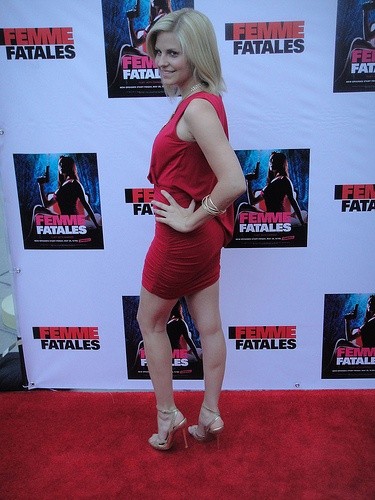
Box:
[202,195,226,216]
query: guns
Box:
[245,162,260,180]
[37,166,50,183]
[344,304,359,320]
[126,0,140,19]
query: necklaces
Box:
[186,81,204,93]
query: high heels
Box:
[188,404,225,450]
[148,396,192,451]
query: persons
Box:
[235,152,303,224]
[331,295,375,359]
[111,0,172,85]
[31,156,99,228]
[136,305,200,361]
[339,0,375,80]
[138,9,246,451]
